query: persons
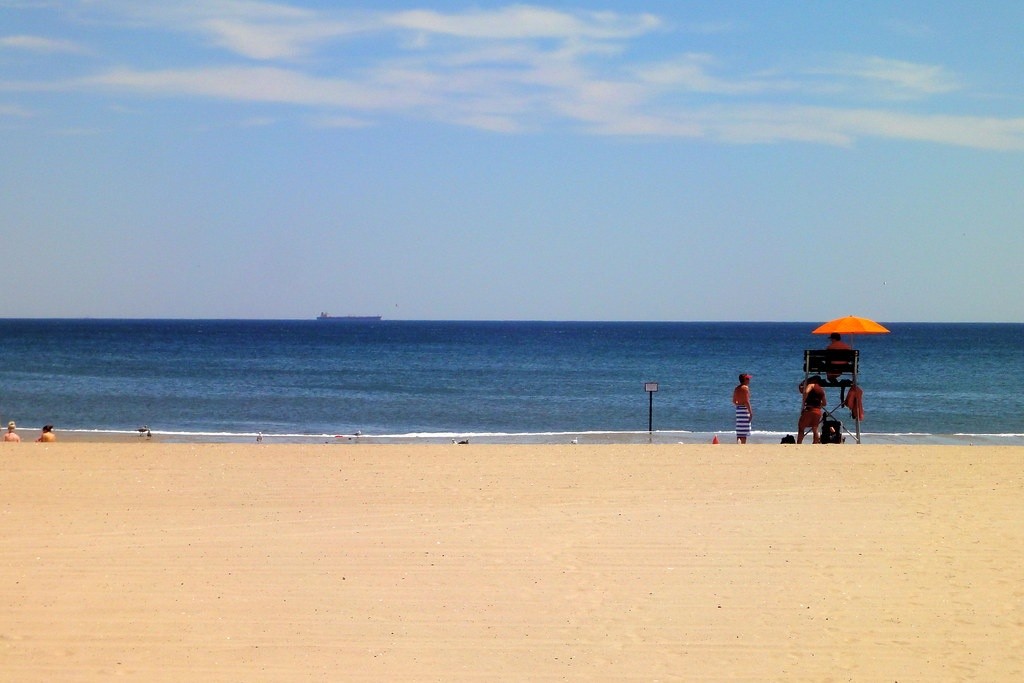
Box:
[796,375,826,444]
[35,425,56,442]
[826,333,853,382]
[3,421,20,443]
[733,373,752,444]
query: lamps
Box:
[646,383,657,391]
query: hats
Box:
[828,333,841,339]
[743,374,752,379]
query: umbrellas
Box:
[812,315,891,345]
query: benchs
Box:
[804,350,860,387]
